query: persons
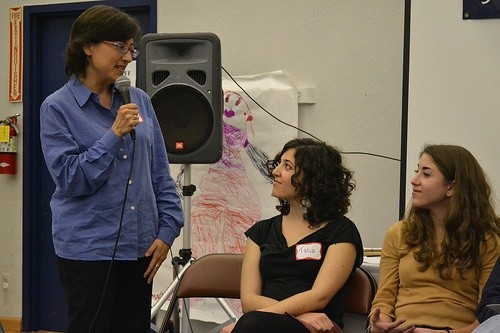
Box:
[221,138,364,333]
[365,145,500,333]
[40,4,184,333]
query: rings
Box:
[135,115,138,120]
[156,264,159,267]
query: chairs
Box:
[158,253,378,333]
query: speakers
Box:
[138,32,223,165]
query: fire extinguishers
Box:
[0,114,21,174]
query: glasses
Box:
[102,40,138,56]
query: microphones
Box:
[115,75,137,141]
